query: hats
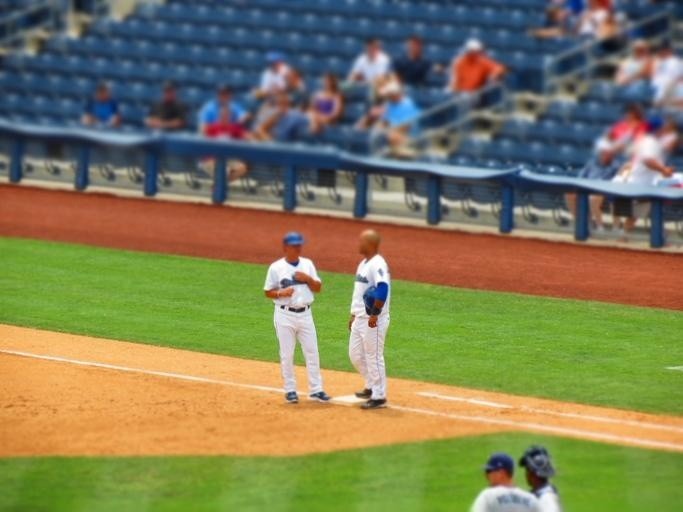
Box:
[282,232,304,245]
[481,452,514,471]
[591,137,617,155]
[378,82,401,96]
[363,286,382,315]
[159,79,182,92]
[518,444,556,478]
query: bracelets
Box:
[368,305,381,315]
[276,289,281,298]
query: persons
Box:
[262,232,331,403]
[572,1,625,37]
[517,445,561,512]
[249,30,508,156]
[346,230,392,410]
[141,77,188,128]
[563,38,681,237]
[525,0,572,40]
[77,83,119,132]
[196,85,252,184]
[468,452,541,512]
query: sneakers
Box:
[285,391,298,403]
[355,389,372,399]
[360,399,387,409]
[308,391,331,403]
